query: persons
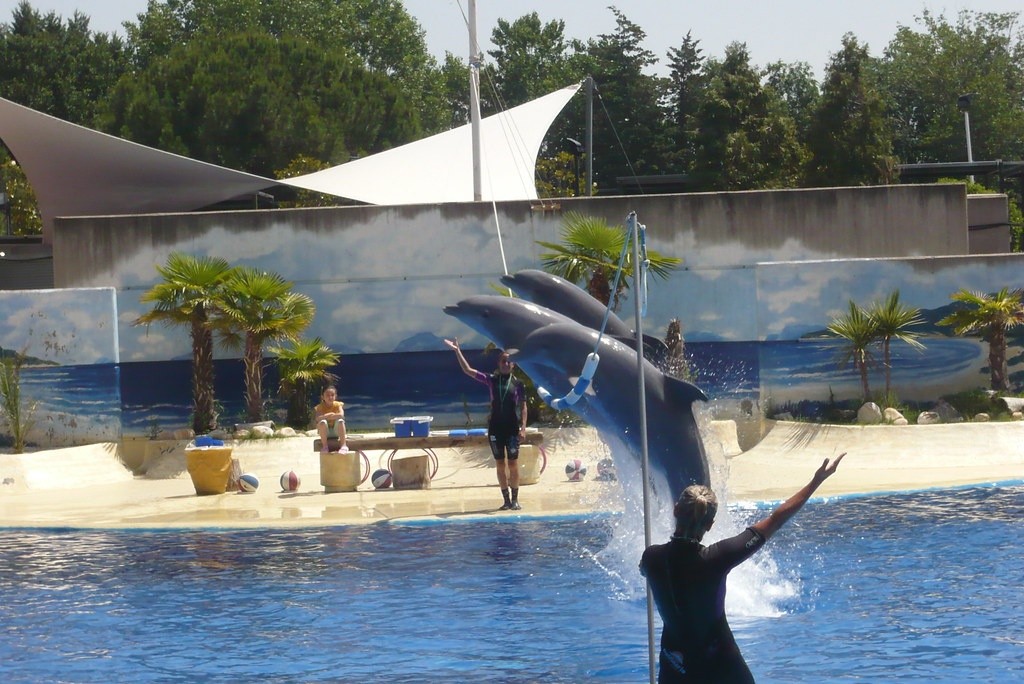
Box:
[314,387,350,454]
[639,454,846,684]
[445,336,527,510]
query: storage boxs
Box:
[390,415,433,438]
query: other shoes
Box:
[338,446,349,455]
[510,502,521,509]
[321,448,329,454]
[500,503,514,510]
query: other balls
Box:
[565,459,586,481]
[280,470,302,491]
[372,469,392,488]
[597,458,616,478]
[238,473,259,492]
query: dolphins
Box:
[443,270,713,532]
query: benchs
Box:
[313,431,543,493]
